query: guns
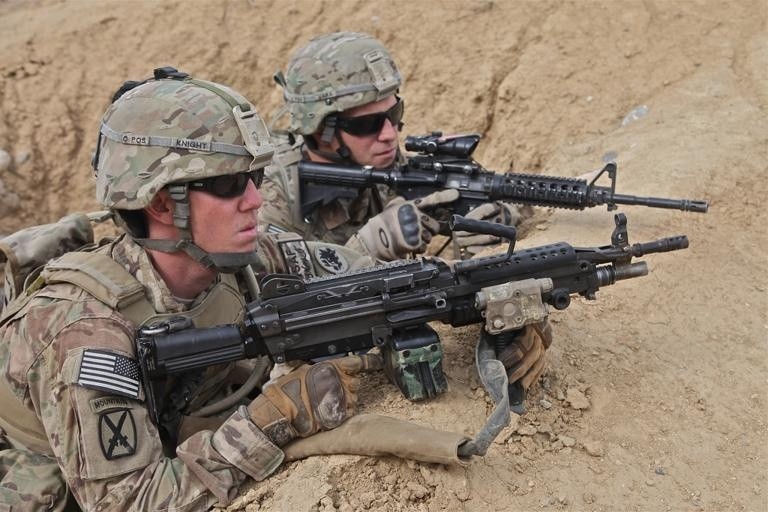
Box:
[298,131,709,234]
[133,214,689,460]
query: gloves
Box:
[343,188,460,262]
[245,351,384,450]
[497,316,555,402]
[457,199,520,256]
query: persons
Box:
[252,32,524,262]
[0,77,554,511]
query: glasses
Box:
[334,93,405,136]
[169,168,263,199]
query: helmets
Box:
[91,66,275,214]
[274,32,403,136]
